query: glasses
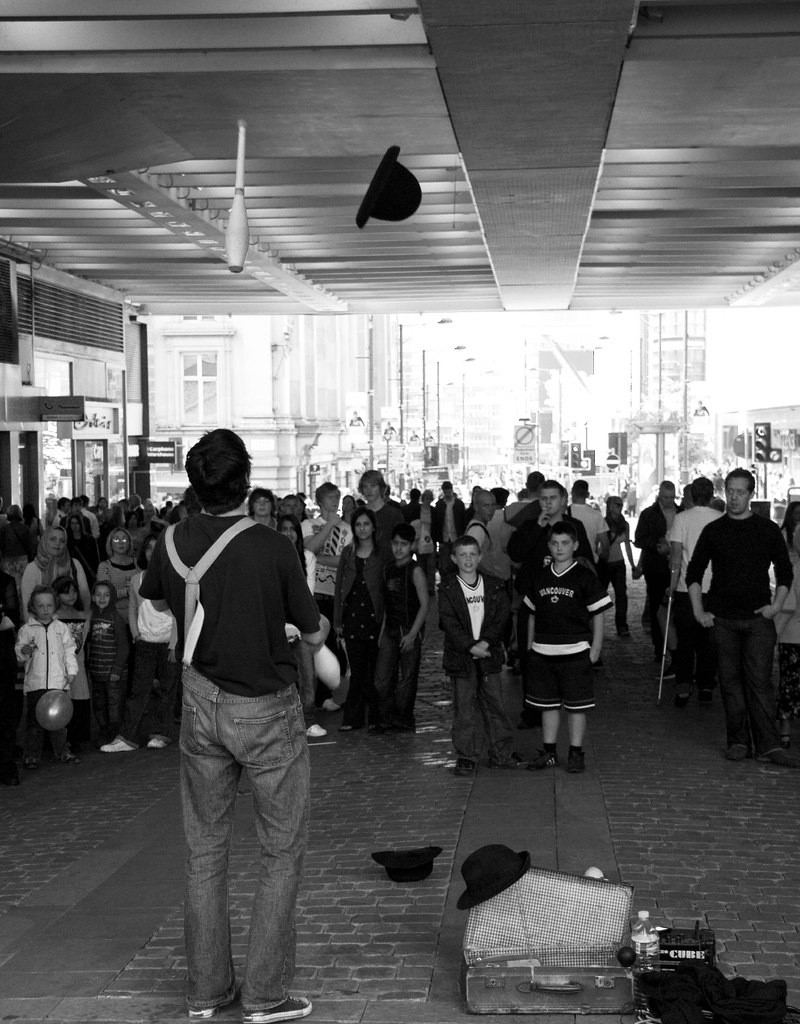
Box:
[111,536,129,544]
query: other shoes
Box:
[755,745,800,768]
[24,754,40,768]
[488,755,521,768]
[615,624,630,637]
[55,750,82,765]
[0,768,20,786]
[337,716,416,737]
[454,757,474,775]
[675,690,689,703]
[778,733,791,748]
[515,718,542,729]
[697,687,714,703]
[511,658,521,675]
[725,743,752,759]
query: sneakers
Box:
[147,738,167,748]
[100,738,136,752]
[529,752,558,770]
[568,748,586,773]
[191,984,236,1018]
[323,699,340,711]
[242,995,313,1023]
[306,723,328,737]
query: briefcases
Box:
[464,867,635,1015]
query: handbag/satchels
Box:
[770,645,780,687]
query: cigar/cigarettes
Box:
[543,508,546,509]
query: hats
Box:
[372,844,442,881]
[456,843,531,910]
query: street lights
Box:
[435,356,476,449]
[397,317,453,495]
[422,345,468,451]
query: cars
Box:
[575,473,631,515]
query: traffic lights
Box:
[558,453,570,468]
[579,458,592,472]
[769,447,781,464]
[570,443,582,468]
[754,422,772,462]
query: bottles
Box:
[632,910,659,1014]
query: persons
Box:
[525,521,614,772]
[0,487,211,785]
[466,472,612,727]
[15,586,83,768]
[684,468,792,760]
[691,465,795,501]
[594,476,727,702]
[247,471,465,736]
[776,501,800,748]
[438,536,519,775]
[137,428,322,1024]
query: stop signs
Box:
[606,455,620,469]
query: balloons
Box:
[36,690,74,730]
